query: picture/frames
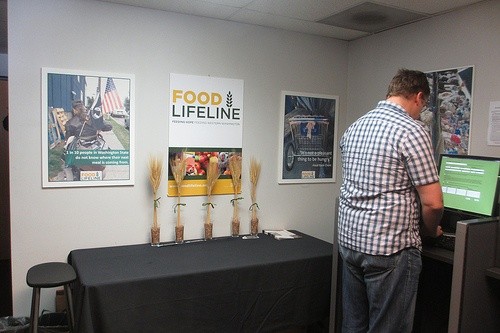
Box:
[277,89,339,184]
[415,65,474,155]
[41,67,134,188]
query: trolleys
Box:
[284,114,333,171]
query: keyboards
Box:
[417,227,456,252]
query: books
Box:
[262,228,303,240]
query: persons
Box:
[63,97,114,181]
[336,69,443,333]
[217,152,229,174]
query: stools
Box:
[26,261,78,333]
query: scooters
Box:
[77,133,108,181]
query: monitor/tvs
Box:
[436,154,500,224]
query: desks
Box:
[67,230,342,333]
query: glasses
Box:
[417,92,429,113]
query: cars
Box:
[125,111,130,129]
[110,108,127,117]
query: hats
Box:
[72,100,82,108]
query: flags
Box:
[100,78,126,114]
